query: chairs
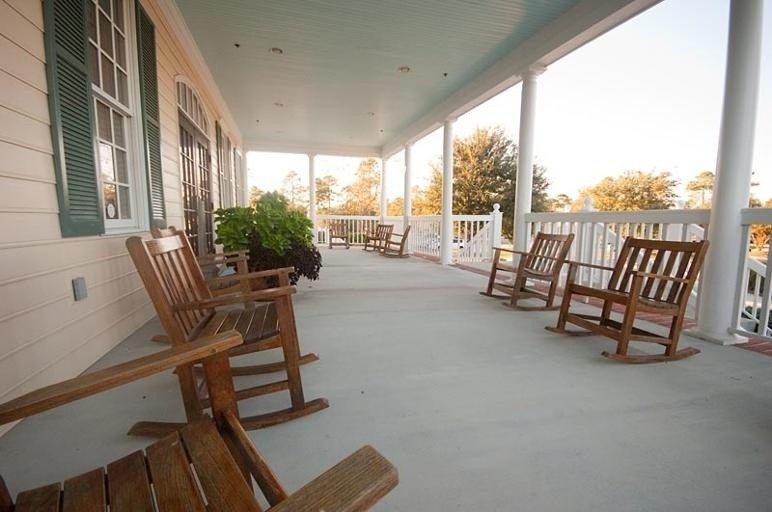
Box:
[543,236,711,363]
[479,232,575,312]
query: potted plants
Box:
[214,190,324,302]
